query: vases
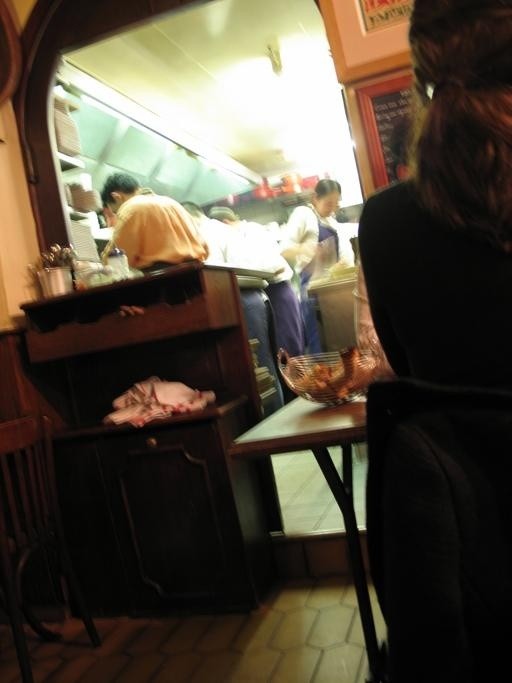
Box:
[35,267,77,301]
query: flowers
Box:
[25,242,73,277]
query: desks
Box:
[231,393,385,683]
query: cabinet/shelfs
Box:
[52,396,282,622]
[51,91,113,272]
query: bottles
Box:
[107,247,130,280]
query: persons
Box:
[349,110,417,350]
[356,0,512,387]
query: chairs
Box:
[0,410,101,683]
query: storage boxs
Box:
[70,219,102,262]
[53,106,82,156]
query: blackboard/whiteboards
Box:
[355,72,414,189]
